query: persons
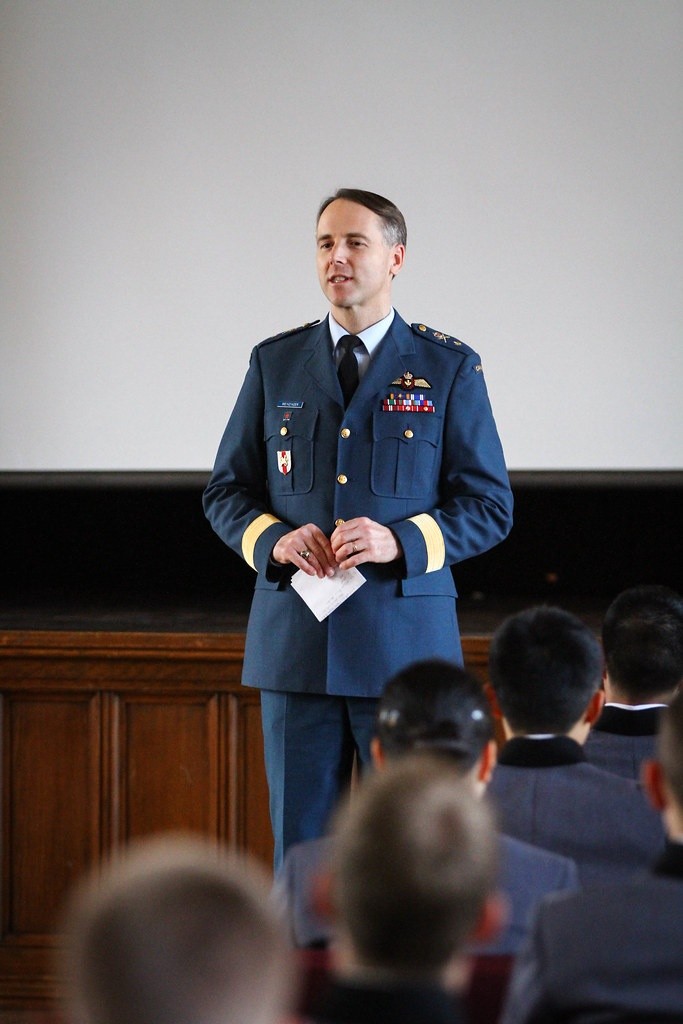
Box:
[201,190,514,867]
[272,756,508,1024]
[483,608,662,871]
[273,658,576,949]
[61,827,279,1024]
[591,590,683,778]
[499,686,683,1024]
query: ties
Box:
[336,331,364,408]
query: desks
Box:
[0,628,507,1024]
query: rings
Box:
[300,548,311,561]
[351,541,358,553]
